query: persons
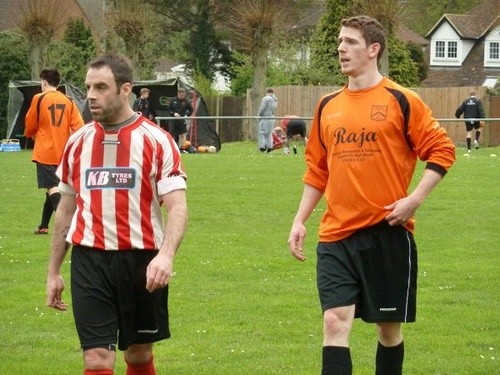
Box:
[257,88,278,153]
[455,90,485,153]
[281,115,308,156]
[272,126,286,149]
[23,68,85,234]
[287,15,457,375]
[132,88,155,122]
[167,88,193,154]
[46,54,188,375]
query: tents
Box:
[82,77,222,154]
[7,80,85,150]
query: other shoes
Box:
[474,140,479,147]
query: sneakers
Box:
[35,228,48,233]
